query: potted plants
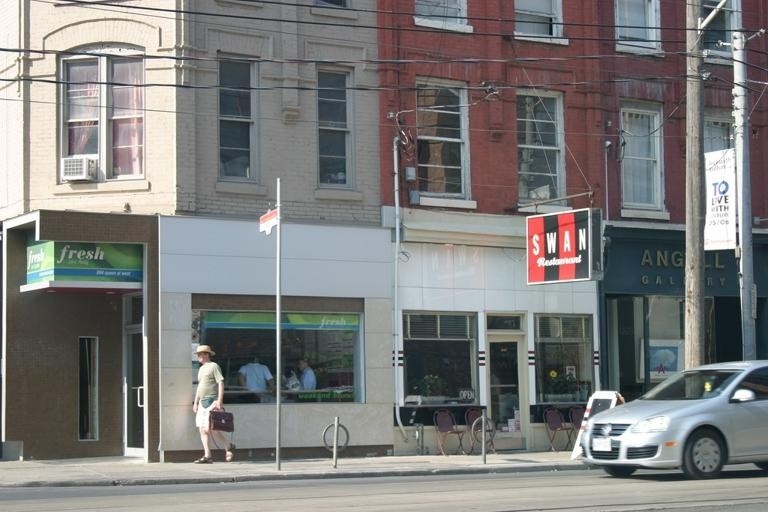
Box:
[543,376,591,402]
[421,374,449,404]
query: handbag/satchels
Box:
[200,396,217,408]
[208,407,234,432]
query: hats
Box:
[192,345,216,356]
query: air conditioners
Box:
[59,154,100,183]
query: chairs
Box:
[542,407,572,451]
[464,407,497,455]
[566,405,587,451]
[433,408,466,455]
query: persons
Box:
[192,345,236,464]
[297,357,317,390]
[238,352,275,395]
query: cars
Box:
[580,359,768,479]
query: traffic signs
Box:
[257,208,278,232]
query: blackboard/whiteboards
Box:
[583,390,620,421]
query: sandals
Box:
[194,456,213,464]
[224,443,236,462]
